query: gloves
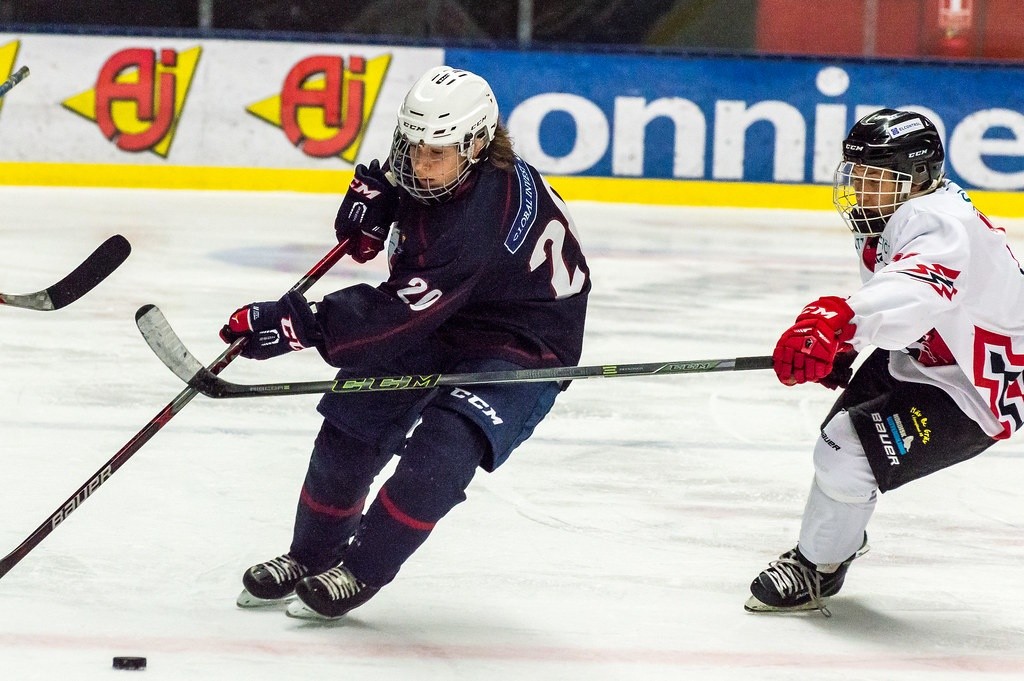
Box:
[773,295,855,385]
[335,162,409,269]
[218,290,317,362]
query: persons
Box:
[744,109,1024,614]
[219,66,591,624]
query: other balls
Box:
[112,655,148,672]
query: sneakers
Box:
[743,525,869,612]
[236,537,342,608]
[285,560,382,621]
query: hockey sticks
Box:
[0,236,355,578]
[133,303,778,401]
[1,233,133,312]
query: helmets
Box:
[387,65,500,204]
[831,107,946,237]
